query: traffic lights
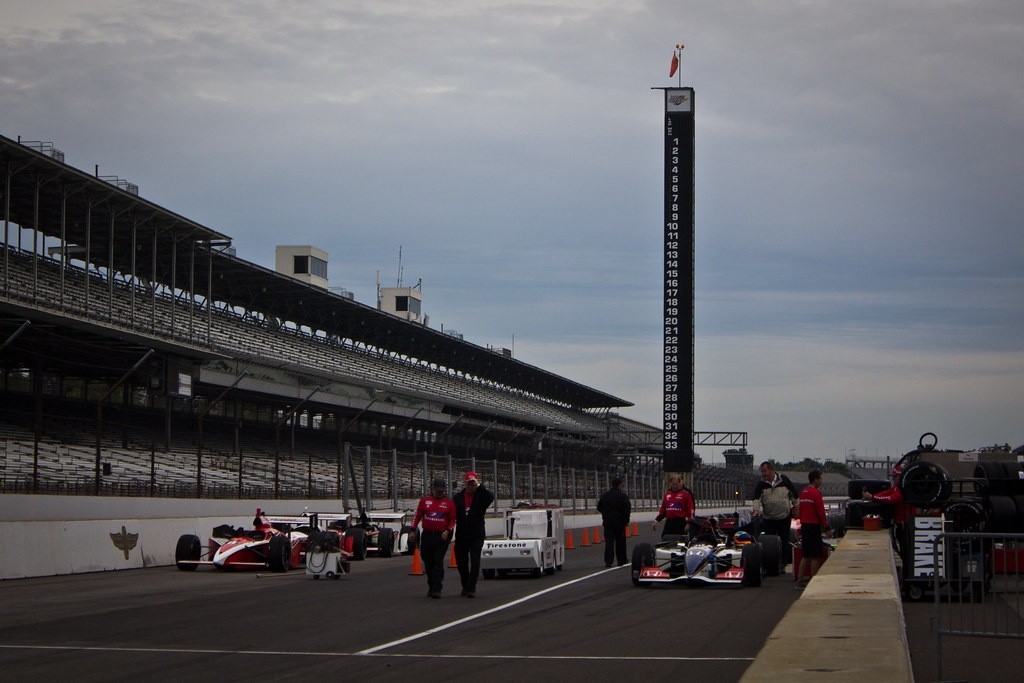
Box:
[736,492,738,495]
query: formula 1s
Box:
[175,507,420,572]
[631,515,783,588]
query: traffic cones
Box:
[592,527,601,544]
[408,547,424,576]
[626,526,631,538]
[565,530,576,549]
[632,519,639,536]
[602,533,606,542]
[579,528,591,547]
[448,542,458,568]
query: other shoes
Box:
[606,564,611,567]
[618,560,629,566]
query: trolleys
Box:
[903,480,993,603]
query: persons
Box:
[751,461,797,565]
[794,470,833,589]
[454,472,493,599]
[408,478,456,598]
[651,475,695,541]
[862,465,917,554]
[597,478,631,567]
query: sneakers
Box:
[467,592,474,597]
[461,588,468,596]
[427,589,432,596]
[794,581,806,590]
[432,591,440,598]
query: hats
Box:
[465,472,478,482]
[434,479,444,488]
[888,466,903,476]
